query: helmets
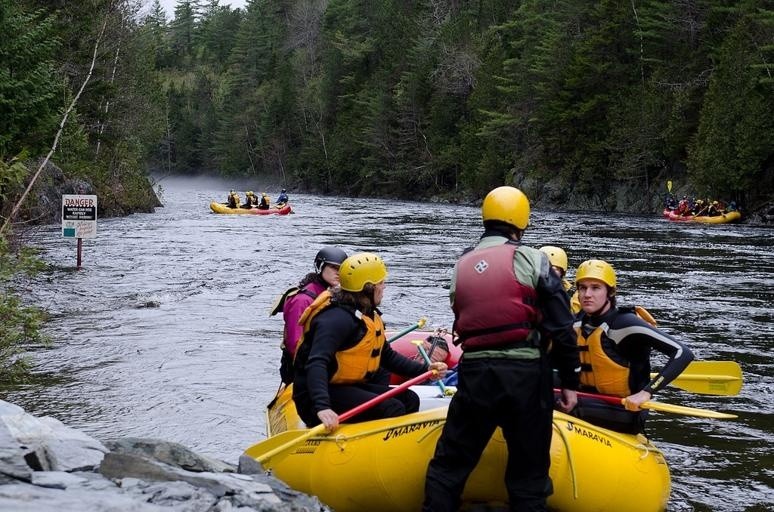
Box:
[669,193,719,205]
[314,246,347,275]
[482,186,530,230]
[576,260,616,288]
[338,253,387,293]
[231,189,286,197]
[538,246,568,272]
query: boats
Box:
[662,205,738,224]
[210,201,289,218]
[266,383,674,512]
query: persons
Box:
[294,253,447,432]
[670,194,738,214]
[554,259,695,436]
[540,245,581,314]
[280,245,348,385]
[424,186,578,512]
[226,187,289,209]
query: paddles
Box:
[651,360,742,397]
[242,370,438,468]
[552,388,737,418]
[668,181,672,193]
